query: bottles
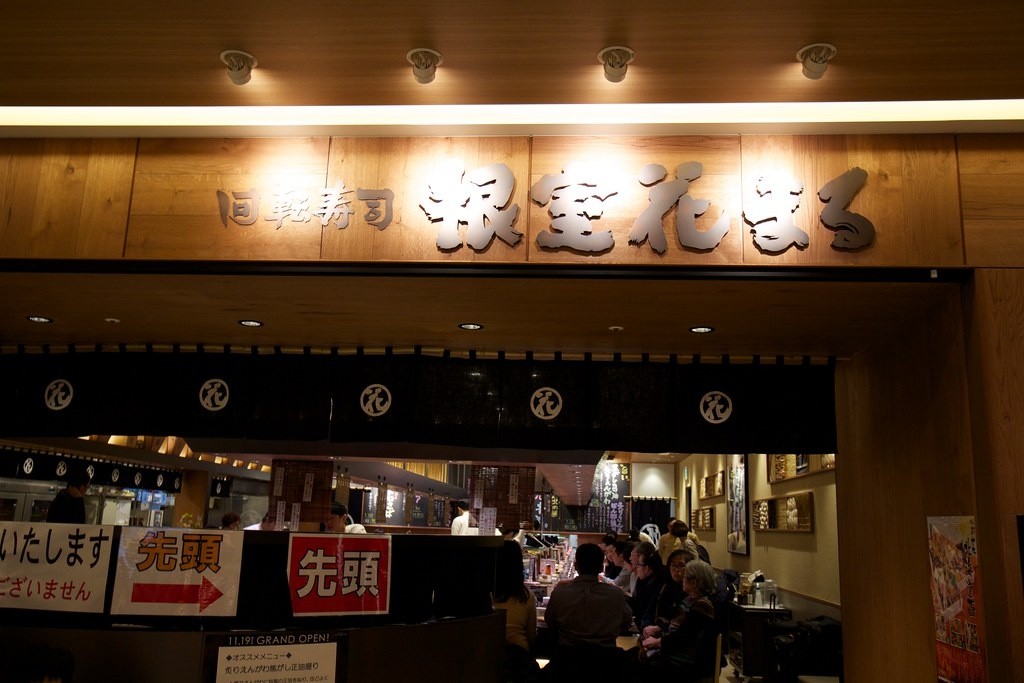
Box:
[763,579,778,605]
[755,587,763,606]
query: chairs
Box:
[704,630,724,683]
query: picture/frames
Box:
[696,469,726,501]
[724,458,749,558]
[689,504,718,532]
[751,490,818,536]
[765,454,835,486]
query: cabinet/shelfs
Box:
[728,601,795,678]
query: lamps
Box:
[793,39,839,82]
[402,45,446,86]
[594,42,637,86]
[214,41,260,90]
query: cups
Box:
[747,594,753,604]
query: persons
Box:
[449,501,719,683]
[222,502,366,534]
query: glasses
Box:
[631,552,642,557]
[636,562,648,567]
